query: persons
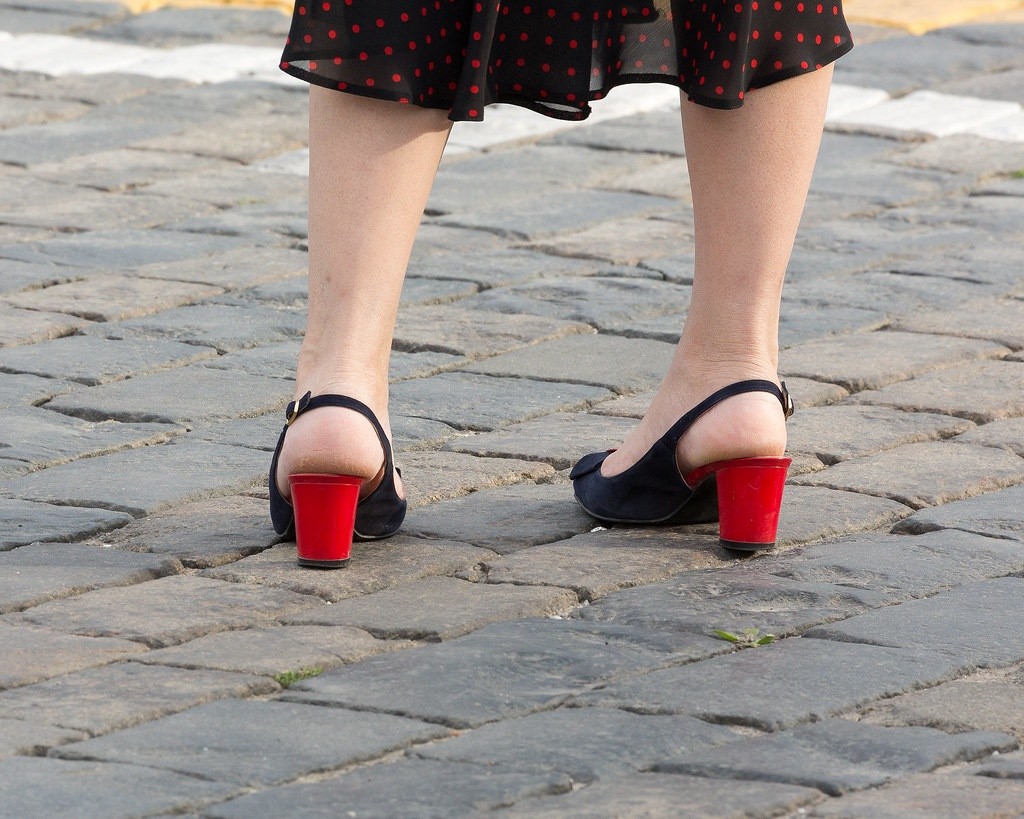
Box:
[269,1,855,570]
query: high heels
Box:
[569,380,796,552]
[269,391,406,569]
